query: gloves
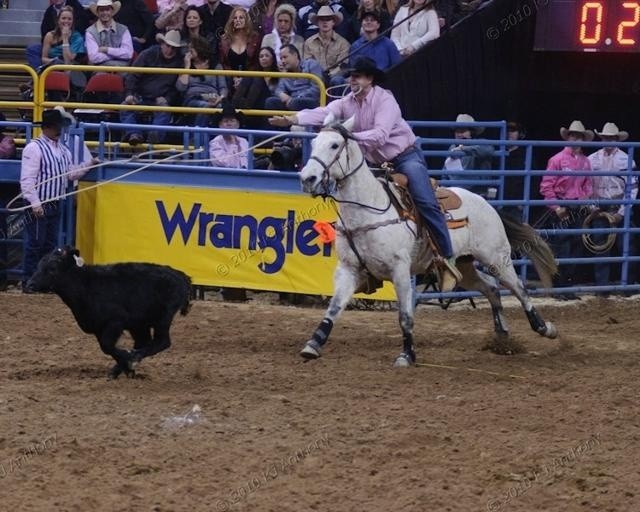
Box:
[268,112,298,127]
[330,123,357,140]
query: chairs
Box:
[33,71,125,140]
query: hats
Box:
[593,122,628,141]
[310,5,344,26]
[210,104,247,128]
[89,0,122,16]
[559,120,595,141]
[32,110,72,126]
[156,30,188,47]
[451,113,485,137]
[342,55,385,84]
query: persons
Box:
[206,102,250,302]
[588,121,638,292]
[267,54,455,267]
[439,112,495,202]
[19,109,103,290]
[23,0,466,149]
[540,120,610,301]
[1,112,17,291]
[496,115,548,200]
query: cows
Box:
[23,244,193,380]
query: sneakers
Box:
[128,134,144,145]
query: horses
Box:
[299,110,560,369]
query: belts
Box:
[390,143,416,162]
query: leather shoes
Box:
[440,256,458,292]
